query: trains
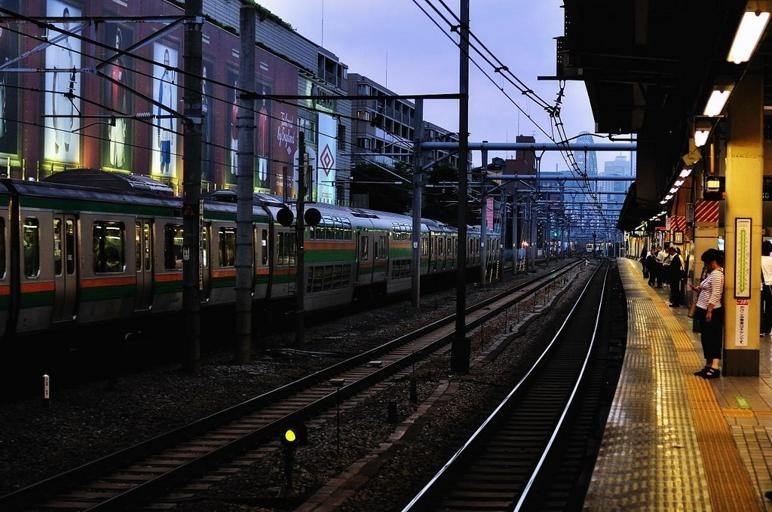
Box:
[1,147,500,388]
[585,242,611,253]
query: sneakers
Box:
[693,365,721,378]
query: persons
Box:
[687,248,724,380]
[48,5,77,162]
[226,77,240,184]
[18,200,244,296]
[156,48,175,177]
[638,244,710,309]
[105,25,129,171]
[1,12,13,146]
[760,240,772,337]
[256,84,271,186]
[199,65,214,185]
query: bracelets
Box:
[707,309,712,312]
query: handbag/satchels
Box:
[763,283,772,302]
